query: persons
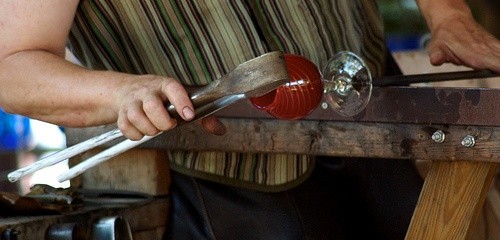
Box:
[0,0,500,240]
